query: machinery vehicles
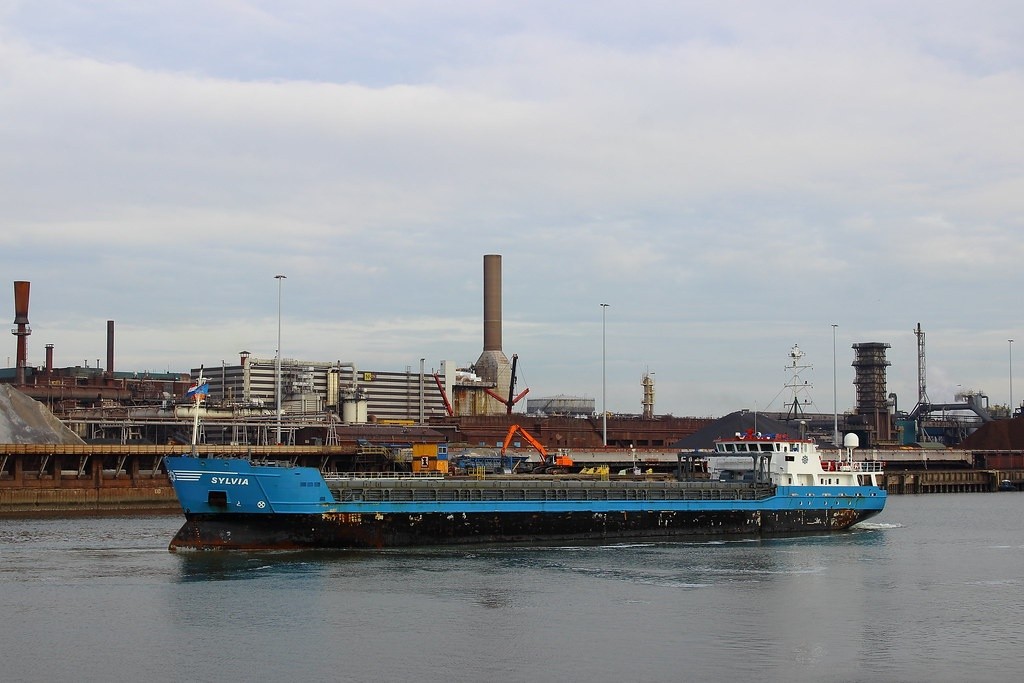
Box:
[494,422,574,475]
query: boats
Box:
[163,342,887,557]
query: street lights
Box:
[829,324,839,447]
[1008,339,1015,419]
[273,274,288,446]
[599,303,611,448]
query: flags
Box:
[184,382,198,399]
[194,383,209,395]
